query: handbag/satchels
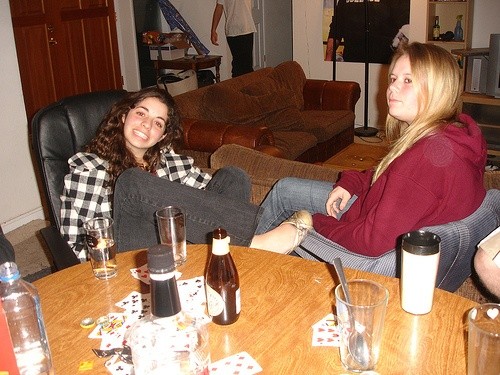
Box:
[157,69,199,97]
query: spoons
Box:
[333,256,369,367]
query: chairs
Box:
[290,189,500,293]
[31,89,131,270]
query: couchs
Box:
[202,144,340,205]
[174,61,361,169]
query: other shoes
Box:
[279,210,313,255]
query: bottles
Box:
[204,228,242,325]
[146,244,182,318]
[432,15,440,41]
[454,15,463,41]
[0,261,55,375]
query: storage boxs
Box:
[149,44,184,61]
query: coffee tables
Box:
[324,142,393,170]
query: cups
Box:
[156,205,187,268]
[467,302,500,375]
[334,279,389,374]
[400,230,442,315]
[83,216,119,281]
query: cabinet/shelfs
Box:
[461,91,500,156]
[425,0,474,92]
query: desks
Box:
[31,243,500,374]
[154,55,223,83]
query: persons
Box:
[60,87,313,261]
[256,43,487,256]
[211,0,257,77]
[326,0,396,63]
